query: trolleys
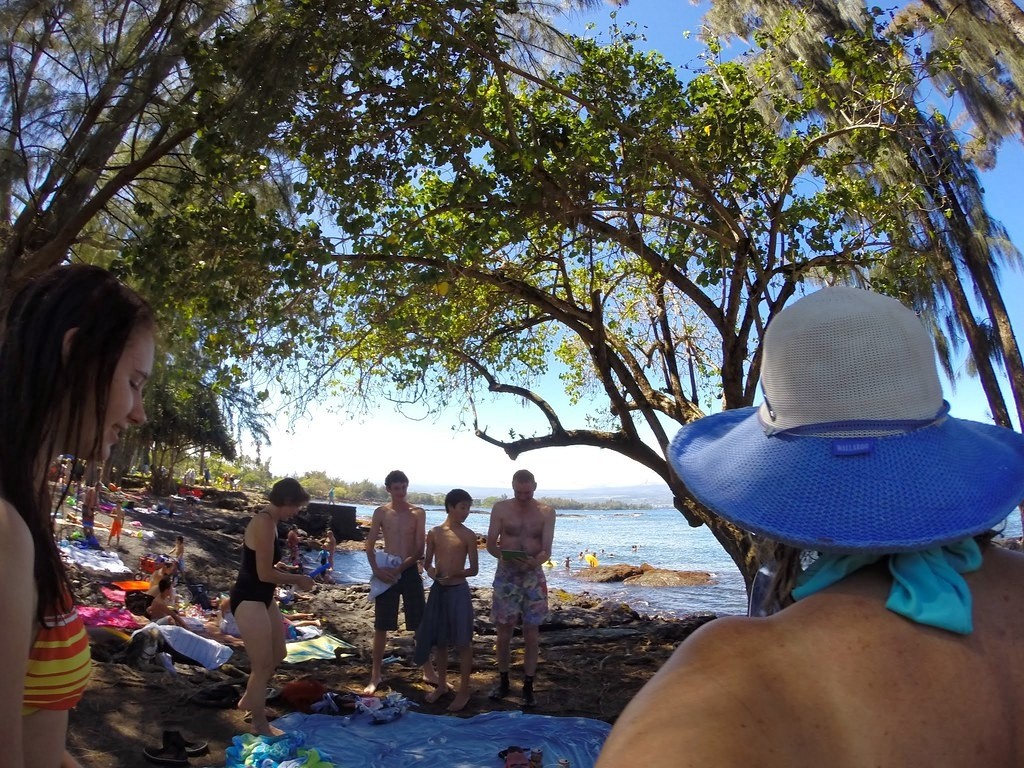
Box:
[134,555,179,583]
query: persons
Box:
[0,265,638,768]
[579,290,1024,768]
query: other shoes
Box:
[520,687,536,707]
[488,685,509,699]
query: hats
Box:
[664,287,1024,553]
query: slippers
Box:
[163,730,208,753]
[143,734,188,763]
